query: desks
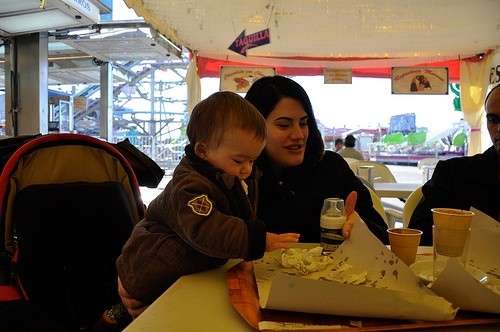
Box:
[372,182,421,200]
[372,182,422,202]
[423,165,437,180]
[120,244,500,332]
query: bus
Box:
[368,141,446,163]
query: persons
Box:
[118,75,391,321]
[407,83,500,247]
[373,138,378,143]
[335,135,363,161]
[118,90,300,321]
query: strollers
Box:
[1,133,148,332]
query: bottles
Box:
[319,198,347,256]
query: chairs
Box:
[350,161,396,182]
[384,187,423,228]
[418,158,439,181]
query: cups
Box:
[387,228,423,267]
[357,165,374,186]
[431,207,474,281]
